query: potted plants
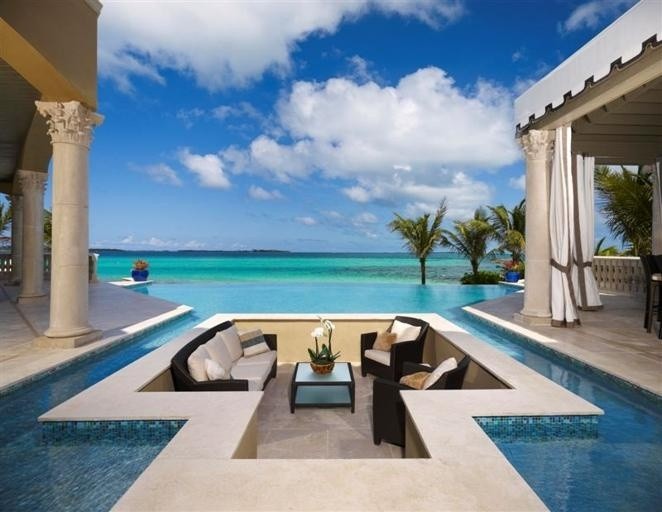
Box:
[504,261,520,281]
[130,258,149,281]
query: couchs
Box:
[170,320,280,392]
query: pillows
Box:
[400,371,433,390]
[203,356,231,381]
[372,332,395,352]
[239,329,269,357]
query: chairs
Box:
[361,314,430,379]
[372,355,470,447]
[639,248,662,338]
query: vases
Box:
[311,363,335,374]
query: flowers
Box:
[307,319,341,364]
